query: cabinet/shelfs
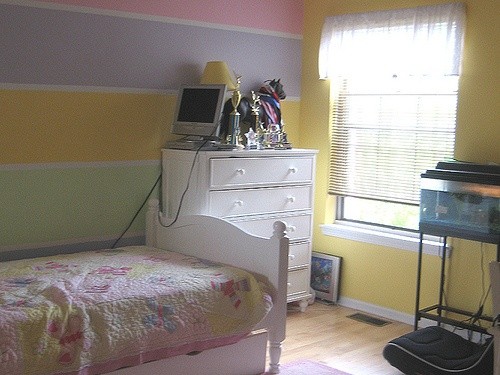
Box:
[160,148,319,312]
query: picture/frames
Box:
[310,250,342,304]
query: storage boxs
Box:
[420,161,500,235]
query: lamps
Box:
[199,61,236,90]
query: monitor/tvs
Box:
[166,83,226,150]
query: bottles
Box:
[247,120,266,150]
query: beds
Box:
[0,197,290,375]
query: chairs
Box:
[383,314,500,375]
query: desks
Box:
[414,222,500,337]
